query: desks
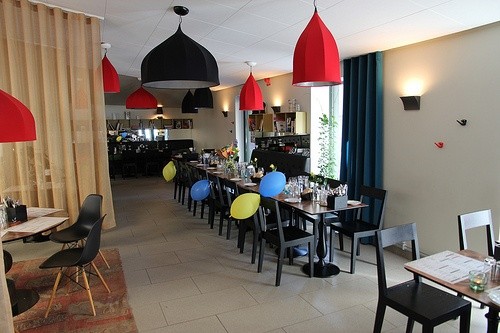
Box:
[186,161,369,278]
[1,210,69,317]
[404,248,500,333]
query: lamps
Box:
[100,5,220,119]
[222,111,228,117]
[400,96,420,110]
[271,106,280,113]
[0,89,36,143]
[292,0,343,87]
[240,61,265,111]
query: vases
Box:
[312,184,318,201]
[225,160,238,178]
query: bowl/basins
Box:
[250,177,261,183]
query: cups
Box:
[0,204,9,229]
[469,270,486,292]
[197,148,264,180]
[7,207,17,223]
[285,176,348,210]
[16,205,28,222]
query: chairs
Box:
[453,209,495,319]
[373,223,471,333]
[171,149,388,287]
[3,194,110,318]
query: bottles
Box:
[209,151,214,167]
[484,241,500,281]
[213,152,218,167]
[201,148,205,164]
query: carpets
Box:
[6,248,138,333]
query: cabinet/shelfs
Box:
[276,112,307,134]
[249,114,273,132]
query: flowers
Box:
[250,157,258,168]
[310,173,322,183]
[217,139,240,170]
[269,164,277,172]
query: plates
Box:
[207,167,217,170]
[197,164,207,167]
[244,183,257,187]
[212,172,224,175]
[229,178,242,182]
[347,200,361,206]
[190,160,197,163]
